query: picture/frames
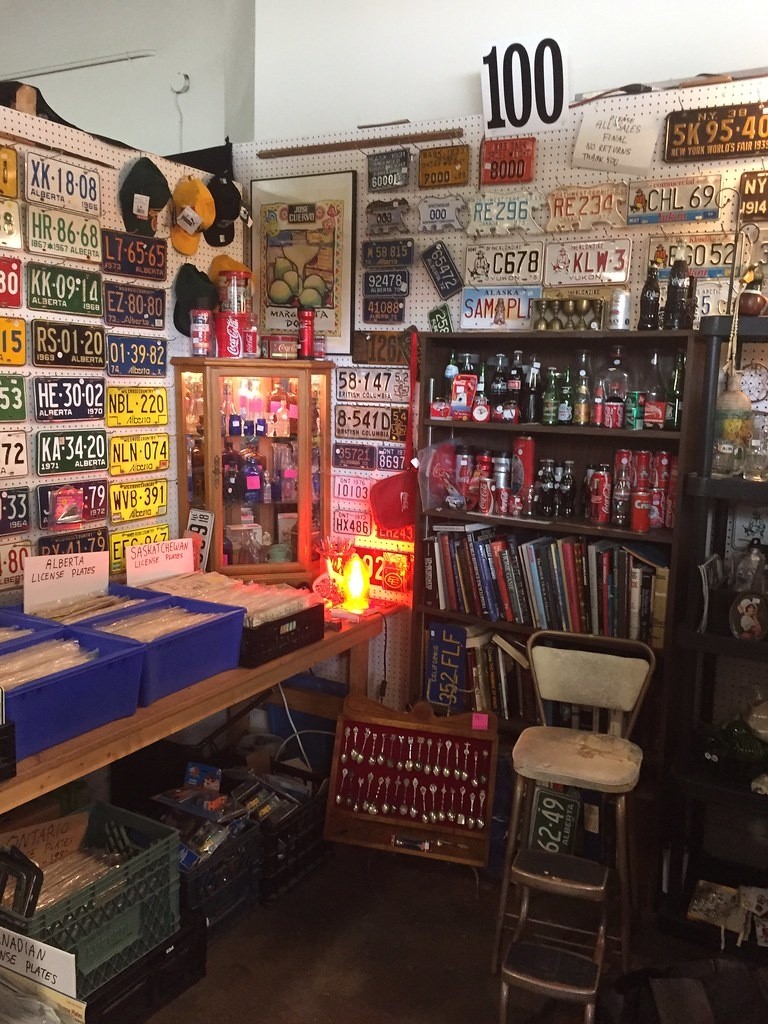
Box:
[251,170,356,355]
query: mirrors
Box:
[171,355,335,584]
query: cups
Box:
[268,544,293,562]
[710,438,768,483]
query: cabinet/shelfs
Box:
[415,329,708,874]
[656,314,768,968]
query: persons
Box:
[737,598,762,640]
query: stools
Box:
[492,630,656,1024]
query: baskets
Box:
[240,599,328,670]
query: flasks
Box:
[296,305,315,359]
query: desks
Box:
[0,595,405,814]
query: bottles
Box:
[638,239,698,329]
[439,344,686,432]
[223,528,233,565]
[191,378,298,505]
[708,375,752,475]
[449,445,632,529]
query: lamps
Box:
[326,551,375,613]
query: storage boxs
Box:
[277,511,298,543]
[0,581,325,781]
[225,523,263,564]
[0,747,334,1024]
[324,712,499,867]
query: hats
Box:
[170,173,216,256]
[174,263,221,336]
[202,173,241,245]
[209,254,253,289]
[121,159,170,237]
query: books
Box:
[420,621,631,741]
[536,779,614,863]
[423,520,669,651]
